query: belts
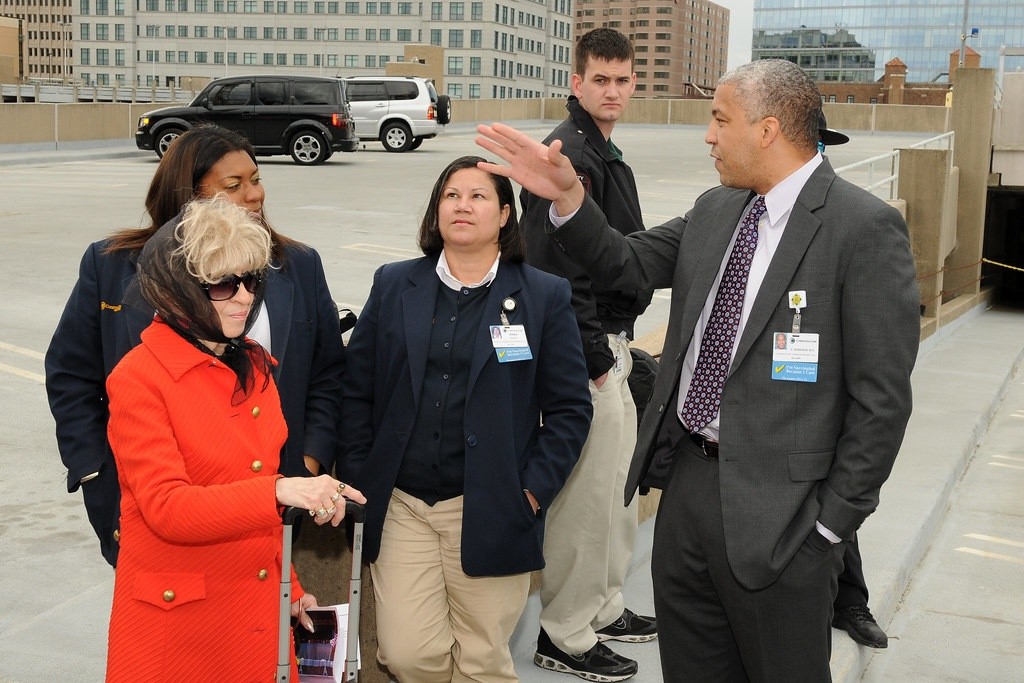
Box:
[684,432,719,461]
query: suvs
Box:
[308,75,452,152]
[135,71,360,165]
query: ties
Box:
[682,195,769,434]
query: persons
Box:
[106,190,367,683]
[775,334,787,349]
[474,59,921,683]
[337,156,594,683]
[518,28,656,682]
[817,111,889,648]
[491,327,502,339]
[41,123,345,571]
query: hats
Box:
[819,111,848,145]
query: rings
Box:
[309,482,346,518]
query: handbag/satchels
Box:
[623,348,661,414]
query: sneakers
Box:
[837,602,889,648]
[533,626,636,682]
[596,609,656,643]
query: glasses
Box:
[203,269,262,301]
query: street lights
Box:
[149,25,161,80]
[57,22,72,80]
[316,26,328,75]
[223,25,234,75]
